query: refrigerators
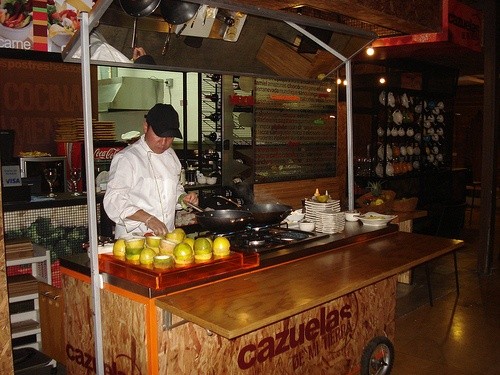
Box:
[56,140,129,193]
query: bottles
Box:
[217,12,236,26]
[204,132,222,142]
[204,113,221,122]
[206,74,240,90]
[203,151,222,160]
[204,94,222,102]
[180,165,196,186]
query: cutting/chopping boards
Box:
[173,5,219,38]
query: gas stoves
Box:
[182,219,330,255]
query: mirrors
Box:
[62,0,377,79]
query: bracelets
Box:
[145,216,155,225]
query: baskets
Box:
[354,183,369,195]
[392,196,417,212]
[356,189,396,212]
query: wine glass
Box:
[43,168,59,198]
[69,168,83,196]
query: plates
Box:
[53,116,117,143]
[222,12,247,43]
[303,198,346,234]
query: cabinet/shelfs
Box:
[234,104,339,184]
[6,244,57,375]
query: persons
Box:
[72,31,156,65]
[104,103,198,241]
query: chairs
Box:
[466,181,481,227]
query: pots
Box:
[211,194,294,226]
[117,0,200,55]
[182,200,254,233]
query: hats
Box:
[144,103,183,139]
[134,55,156,65]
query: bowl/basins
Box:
[48,30,74,48]
[299,222,314,232]
[198,176,218,185]
[345,210,360,221]
[354,211,398,226]
[0,14,33,42]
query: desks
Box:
[156,230,464,340]
[356,211,429,284]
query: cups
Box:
[374,91,445,178]
